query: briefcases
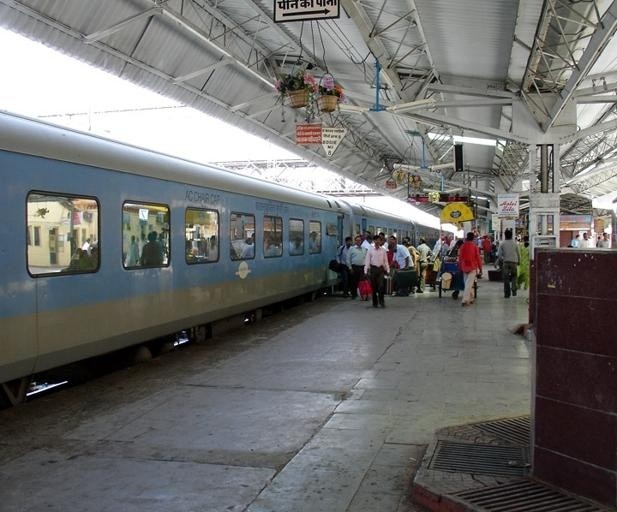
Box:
[398,270,416,287]
[384,275,393,294]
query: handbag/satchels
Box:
[357,280,373,295]
[329,260,344,273]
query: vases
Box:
[283,89,309,108]
[317,96,338,112]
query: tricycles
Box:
[435,251,482,299]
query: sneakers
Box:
[505,289,516,298]
[463,300,475,306]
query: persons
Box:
[569,230,611,249]
[58,225,322,274]
[328,225,532,307]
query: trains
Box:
[0,104,461,405]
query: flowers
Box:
[393,168,441,204]
[314,74,344,116]
[273,66,318,123]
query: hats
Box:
[420,236,425,241]
